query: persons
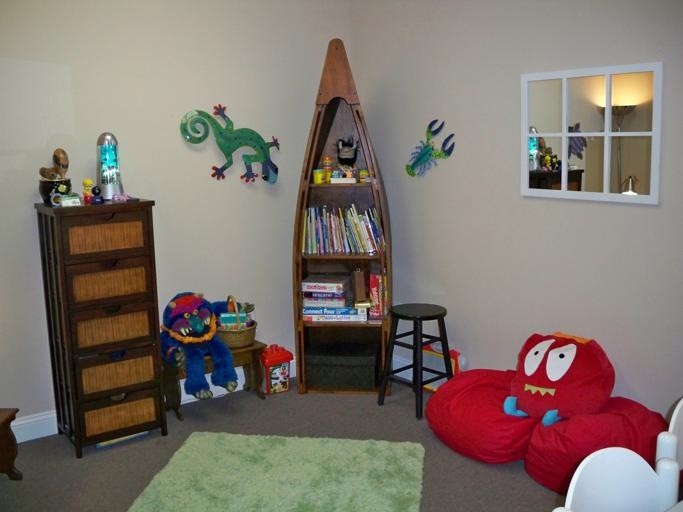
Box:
[81,178,93,204]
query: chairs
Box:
[549,395,681,511]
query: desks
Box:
[165,341,266,421]
[0,407,25,484]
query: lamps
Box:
[594,103,637,195]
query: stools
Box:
[376,304,454,421]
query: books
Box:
[93,431,152,450]
[299,204,387,324]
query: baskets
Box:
[216,295,256,348]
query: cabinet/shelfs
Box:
[292,37,392,394]
[531,169,581,193]
[33,195,167,459]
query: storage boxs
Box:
[256,344,293,396]
[307,350,376,391]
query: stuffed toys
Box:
[159,291,254,400]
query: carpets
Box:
[127,432,427,512]
